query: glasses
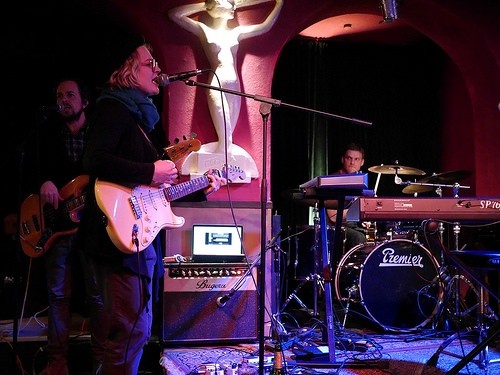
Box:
[140,59,158,72]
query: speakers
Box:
[160,260,262,345]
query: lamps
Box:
[380,0,399,22]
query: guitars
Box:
[93,163,245,254]
[19,132,202,257]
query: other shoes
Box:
[39,356,69,375]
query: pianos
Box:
[346,196,500,316]
[286,172,393,368]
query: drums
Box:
[365,218,415,240]
[334,239,445,334]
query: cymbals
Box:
[402,171,471,194]
[367,165,426,175]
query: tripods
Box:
[434,223,500,330]
[281,202,323,323]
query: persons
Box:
[168,0,283,153]
[327,142,367,258]
[82,32,226,375]
[32,80,92,375]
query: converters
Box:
[340,339,368,351]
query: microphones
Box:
[42,105,62,111]
[216,294,231,308]
[156,68,210,86]
